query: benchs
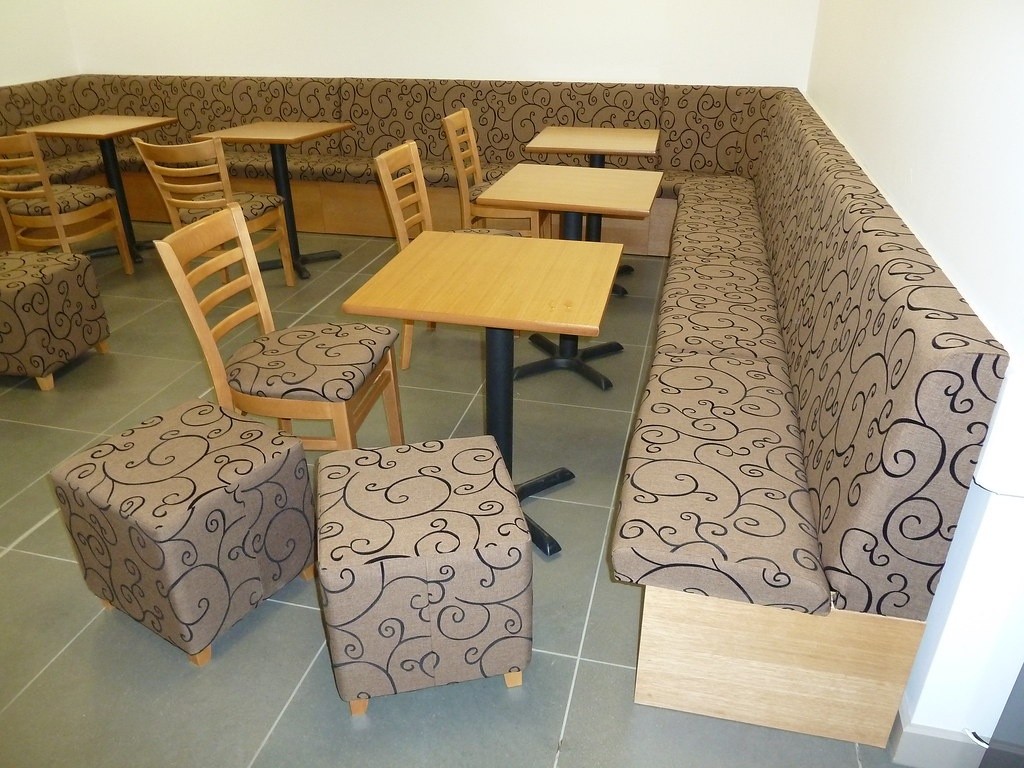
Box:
[1,73,1012,746]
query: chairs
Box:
[0,133,134,274]
[373,139,518,369]
[154,201,404,451]
[133,137,294,288]
[442,106,553,238]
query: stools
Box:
[315,434,534,713]
[0,249,110,393]
[49,400,314,666]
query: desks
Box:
[526,126,660,297]
[343,230,623,554]
[193,121,356,278]
[16,114,178,261]
[476,164,664,389]
